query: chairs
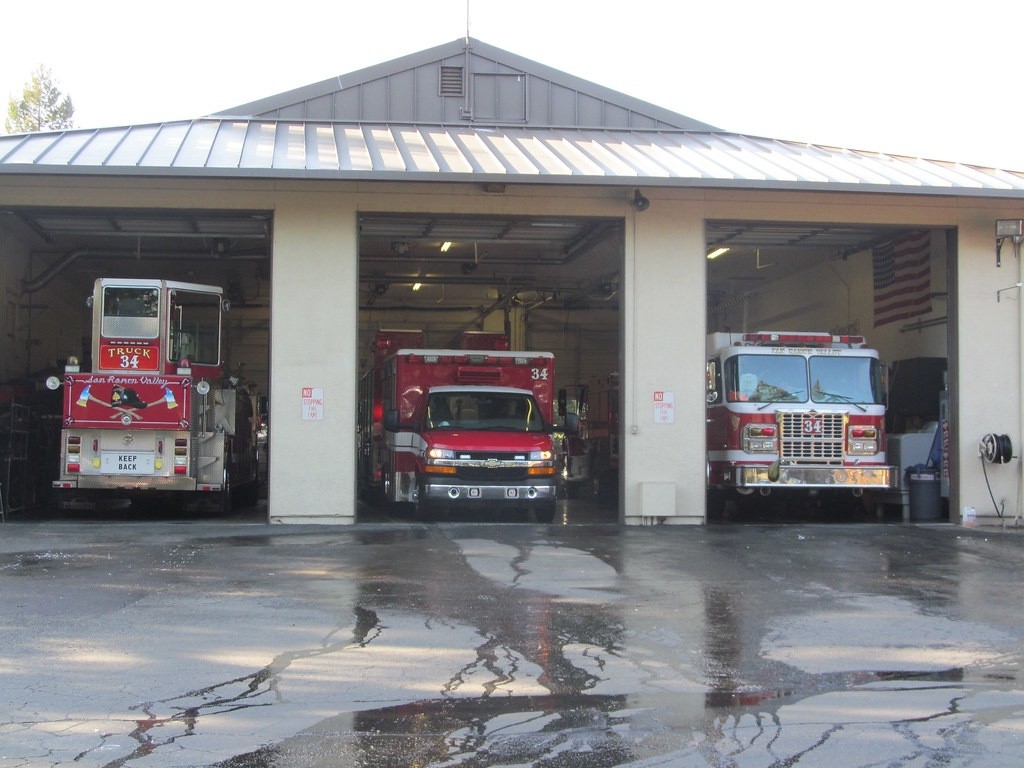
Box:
[740,374,759,394]
[458,409,480,428]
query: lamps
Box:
[995,218,1024,267]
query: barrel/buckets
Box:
[907,468,944,524]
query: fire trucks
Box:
[357,329,579,522]
[609,330,898,516]
[46,278,256,514]
